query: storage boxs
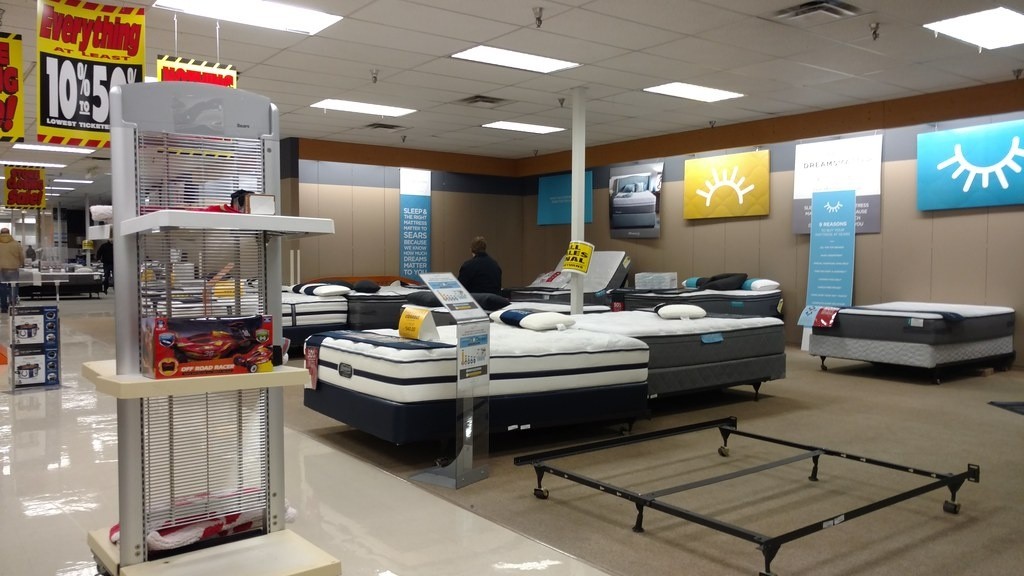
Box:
[138,317,274,379]
[6,305,60,390]
[635,272,678,289]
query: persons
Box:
[458,236,502,295]
[0,228,24,313]
[26,245,36,261]
[95,239,113,295]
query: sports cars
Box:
[173,330,260,361]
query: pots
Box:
[16,322,37,339]
[14,363,41,378]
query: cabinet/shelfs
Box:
[82,80,343,576]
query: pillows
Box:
[654,302,706,318]
[488,308,575,329]
[635,182,643,191]
[743,277,781,291]
[354,280,381,293]
[682,277,709,288]
[287,282,324,291]
[408,290,442,308]
[624,184,635,192]
[470,292,511,311]
[300,284,350,296]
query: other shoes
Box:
[1,308,7,313]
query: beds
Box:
[172,263,787,466]
[809,300,1015,385]
[611,175,657,228]
